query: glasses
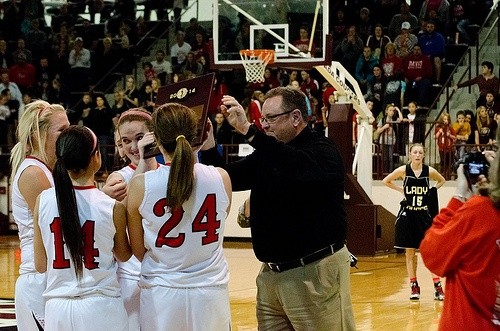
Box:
[259,109,294,125]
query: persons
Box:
[420,147,500,330]
[218,87,357,331]
[10,100,128,330]
[0,0,500,175]
[382,143,447,300]
[107,109,164,183]
[100,114,128,201]
[125,102,234,331]
[32,124,132,331]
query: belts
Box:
[265,240,344,273]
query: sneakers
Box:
[409,283,420,300]
[434,287,445,301]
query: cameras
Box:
[450,152,490,185]
